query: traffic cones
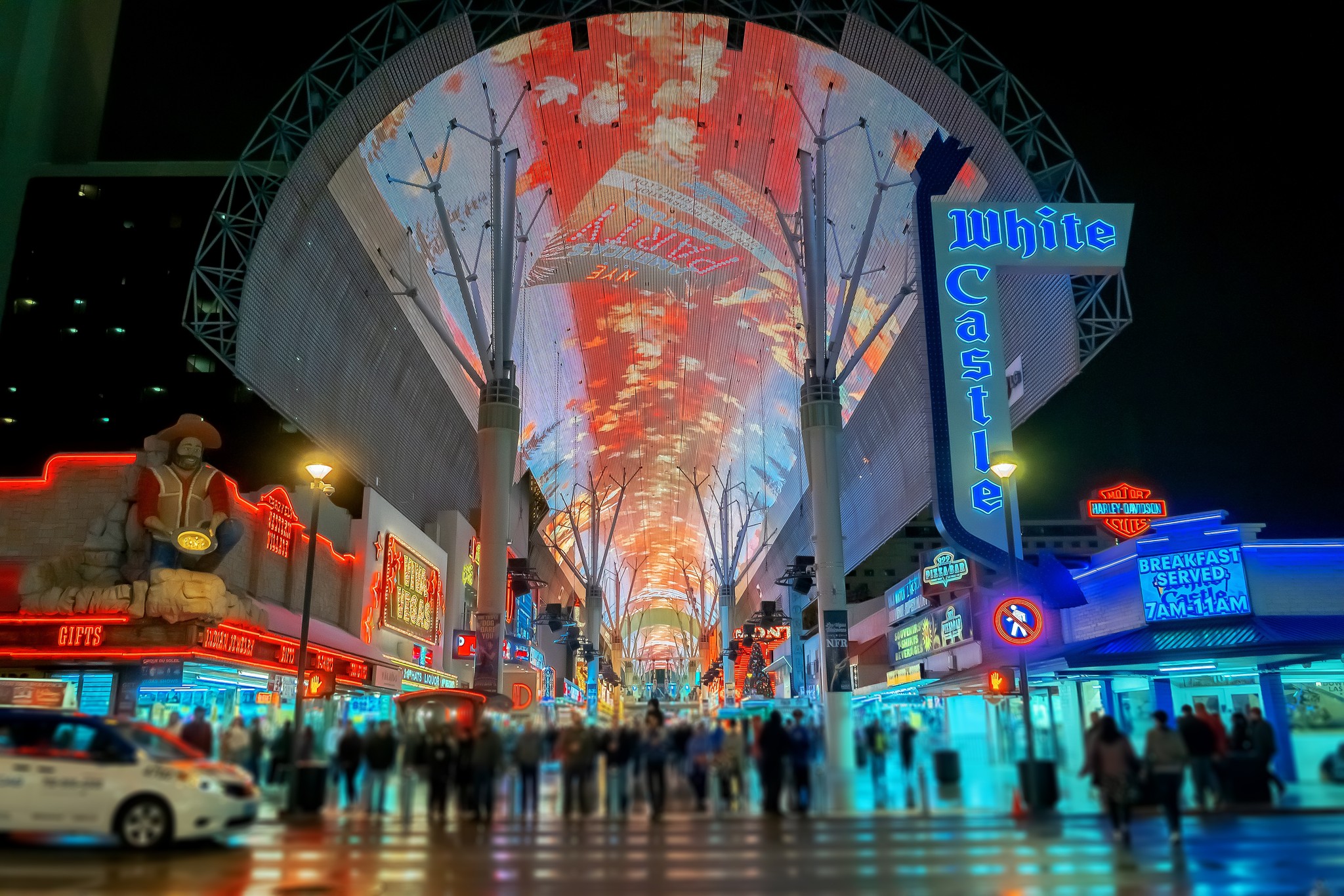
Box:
[1008,788,1028,820]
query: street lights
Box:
[292,452,334,761]
[989,451,1041,819]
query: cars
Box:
[0,703,230,855]
[122,723,264,828]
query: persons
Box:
[156,696,672,823]
[677,708,919,817]
[139,413,244,573]
[1077,701,1290,838]
